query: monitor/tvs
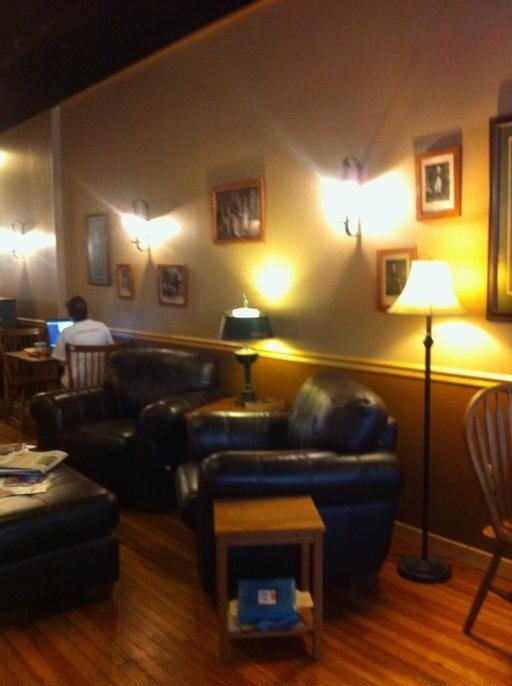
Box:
[44,318,75,349]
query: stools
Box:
[0,441,120,621]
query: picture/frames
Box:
[156,263,189,307]
[413,145,459,222]
[375,247,415,312]
[486,114,512,322]
[210,178,266,244]
[116,264,135,299]
[83,214,112,287]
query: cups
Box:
[34,342,47,358]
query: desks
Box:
[186,394,282,424]
[7,346,60,438]
[212,496,324,664]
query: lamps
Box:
[218,293,273,406]
[321,154,363,238]
[386,260,463,584]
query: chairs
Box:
[175,373,400,615]
[0,325,46,433]
[29,346,215,501]
[65,341,134,390]
[464,383,512,632]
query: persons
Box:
[51,295,114,392]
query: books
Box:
[0,449,69,476]
